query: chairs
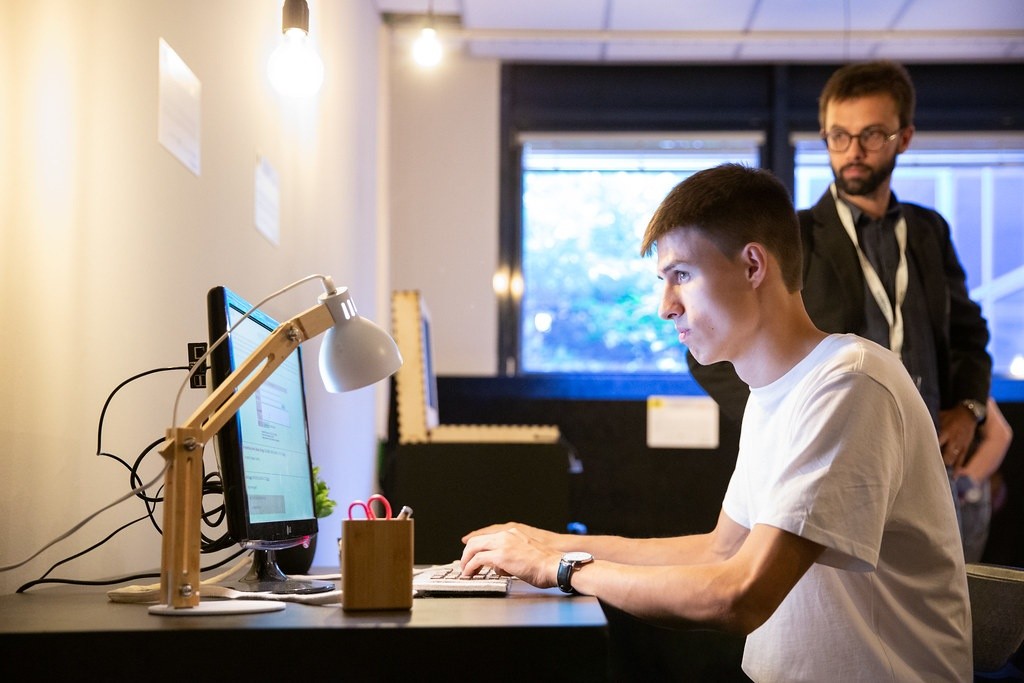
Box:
[966,562,1024,683]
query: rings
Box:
[953,449,959,455]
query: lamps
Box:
[144,271,402,616]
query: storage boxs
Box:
[340,517,413,613]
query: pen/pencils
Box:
[397,505,412,519]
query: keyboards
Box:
[412,560,512,599]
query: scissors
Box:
[349,494,392,521]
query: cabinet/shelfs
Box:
[384,439,591,564]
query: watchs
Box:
[963,400,986,421]
[557,552,594,593]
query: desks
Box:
[0,569,608,683]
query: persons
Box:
[461,164,974,683]
[687,56,1011,564]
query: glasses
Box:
[822,123,908,153]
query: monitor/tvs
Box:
[206,285,335,594]
